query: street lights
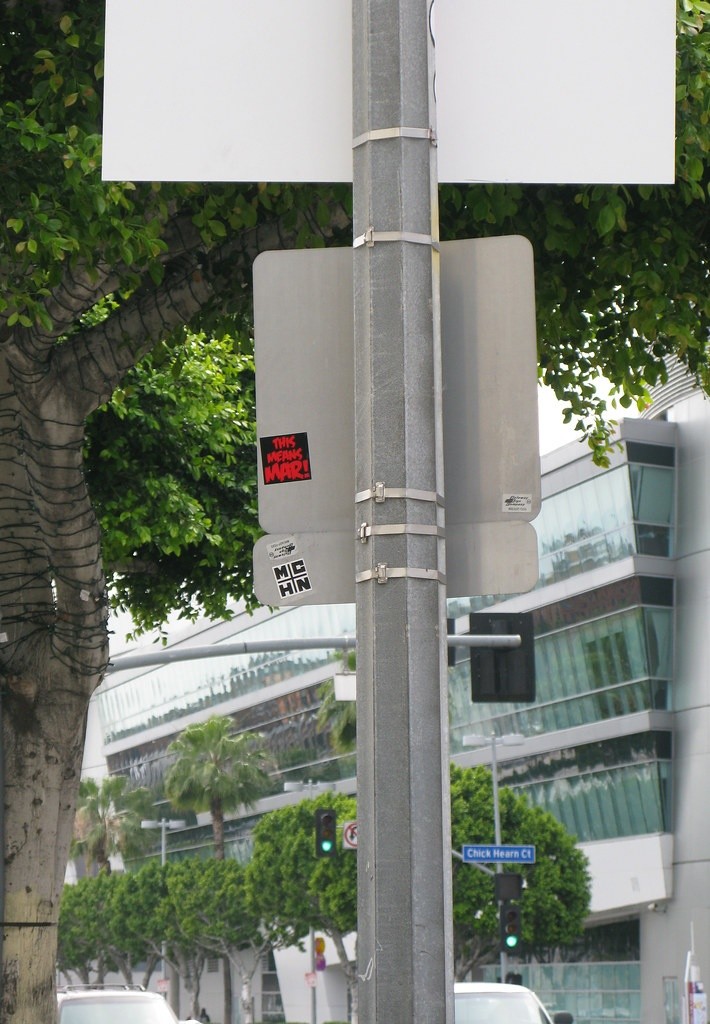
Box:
[463,731,525,983]
[284,779,334,1024]
[141,819,186,999]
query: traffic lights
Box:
[316,809,336,857]
[496,873,523,955]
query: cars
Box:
[454,983,554,1024]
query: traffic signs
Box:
[463,845,536,863]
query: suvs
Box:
[57,984,179,1024]
